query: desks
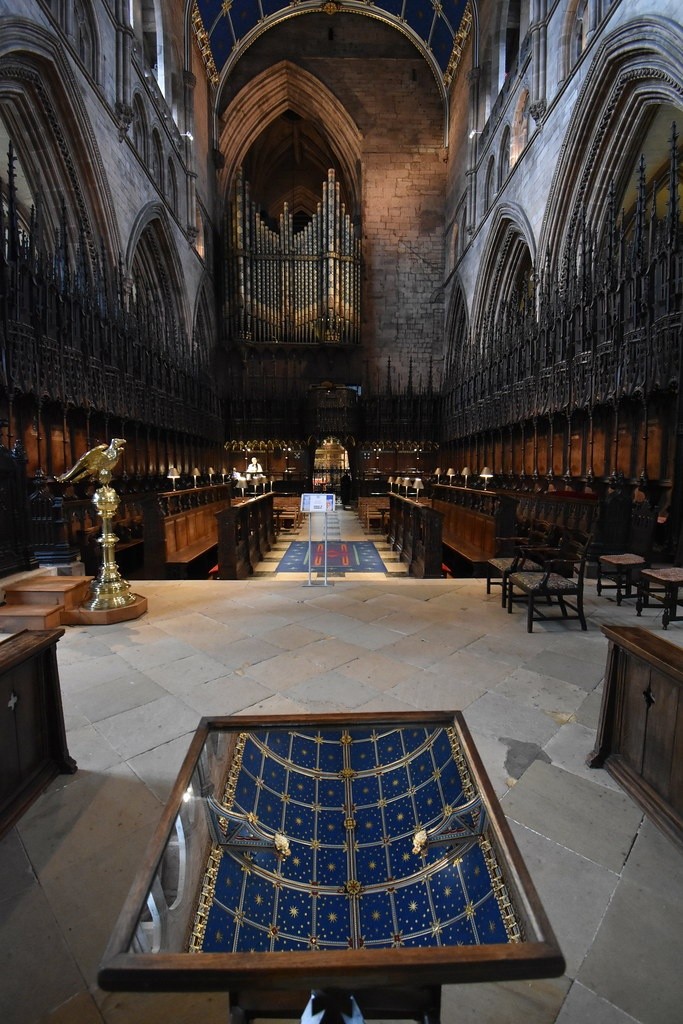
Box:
[97,710,567,1024]
[586,624,682,858]
[0,628,78,843]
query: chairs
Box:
[597,500,657,607]
[486,519,592,633]
[636,524,683,630]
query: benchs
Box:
[430,484,603,578]
[65,483,231,580]
[233,495,307,534]
[357,496,433,531]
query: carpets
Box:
[275,541,388,572]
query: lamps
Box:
[249,476,275,498]
[207,467,215,486]
[435,467,444,484]
[190,467,201,489]
[387,476,424,503]
[235,478,248,503]
[167,468,180,492]
[480,467,493,491]
[461,467,472,489]
[446,468,455,487]
[219,468,227,485]
